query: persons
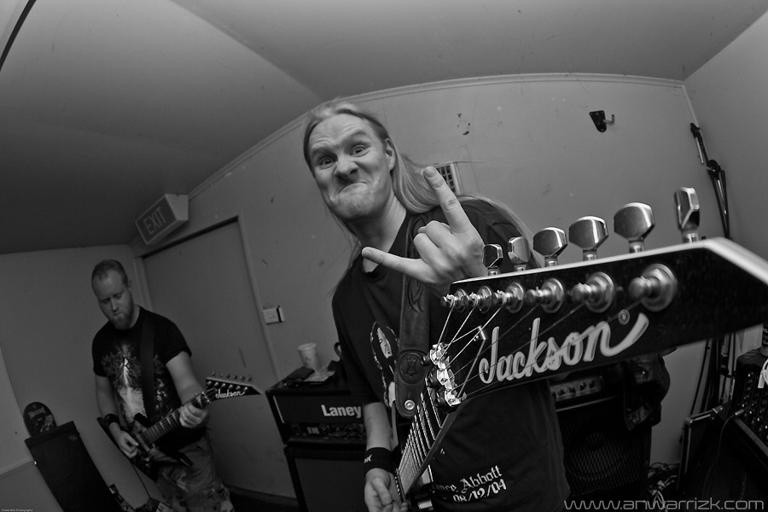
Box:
[305,98,573,512]
[88,262,231,511]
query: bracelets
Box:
[101,411,127,430]
[363,445,395,477]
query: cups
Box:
[296,341,322,371]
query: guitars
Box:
[389,186,768,511]
[124,371,262,478]
